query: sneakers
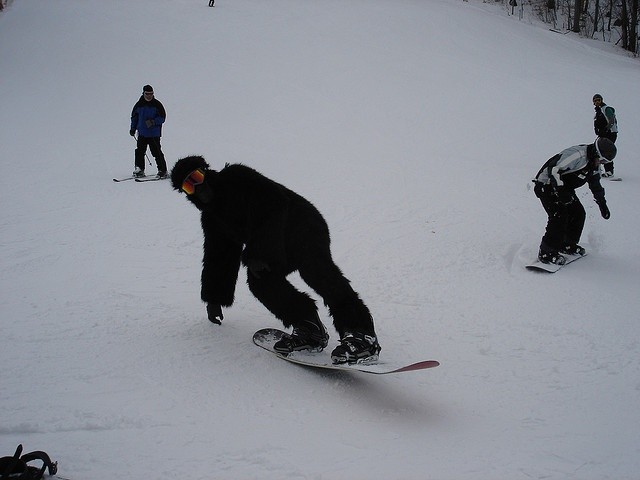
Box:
[601,173,612,177]
[157,169,166,176]
[273,316,329,354]
[133,167,144,177]
[561,244,585,255]
[331,330,381,365]
[538,248,564,266]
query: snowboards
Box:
[253,328,440,374]
[525,249,590,273]
[599,176,622,181]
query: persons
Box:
[592,93,619,177]
[128,84,168,177]
[532,136,617,265]
[168,154,381,364]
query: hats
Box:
[598,138,617,160]
[593,94,603,101]
[142,85,153,94]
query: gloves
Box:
[597,200,610,219]
[558,187,571,203]
[130,129,135,136]
[146,119,155,128]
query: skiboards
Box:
[113,173,172,182]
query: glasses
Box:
[595,136,613,165]
[594,99,601,102]
[181,168,204,195]
[144,91,153,96]
